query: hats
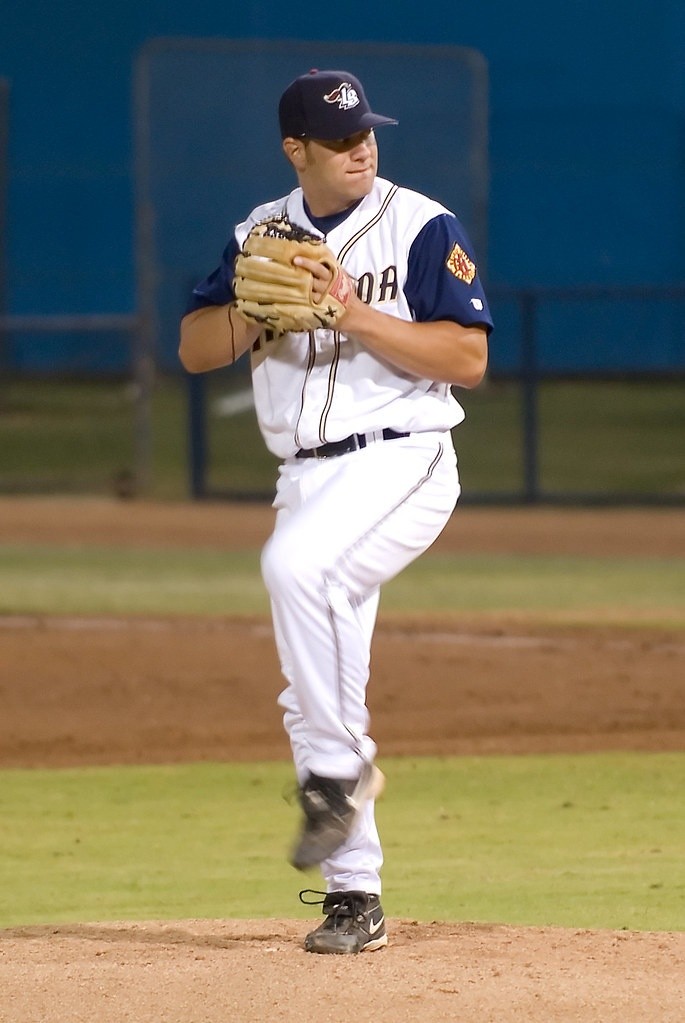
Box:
[278,68,400,141]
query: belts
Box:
[295,427,411,456]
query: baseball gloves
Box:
[231,215,352,334]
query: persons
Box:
[177,69,494,955]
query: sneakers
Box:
[298,888,386,954]
[291,763,384,871]
